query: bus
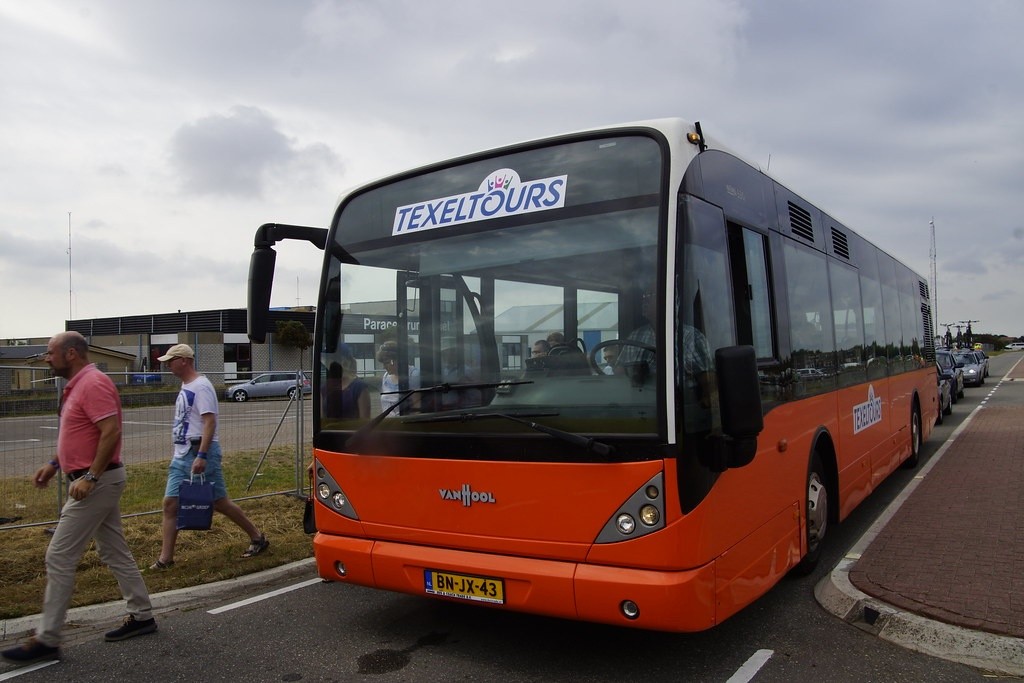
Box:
[246,119,939,636]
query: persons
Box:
[376,341,420,418]
[441,337,477,404]
[612,283,715,431]
[141,344,270,570]
[304,343,371,477]
[531,333,619,377]
[0,331,157,664]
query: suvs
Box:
[225,371,311,402]
[1004,343,1024,351]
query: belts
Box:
[67,461,124,481]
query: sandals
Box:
[240,533,270,558]
[140,560,175,573]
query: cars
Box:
[953,350,989,388]
[935,349,965,406]
[932,362,954,427]
[791,356,923,378]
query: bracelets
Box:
[197,452,208,460]
[48,460,59,469]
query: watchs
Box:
[85,473,98,482]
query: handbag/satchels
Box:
[547,338,586,357]
[304,478,315,534]
[177,472,215,530]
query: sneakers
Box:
[105,615,157,640]
[1,639,59,662]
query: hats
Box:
[321,344,357,368]
[157,344,195,361]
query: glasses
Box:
[168,357,183,361]
[532,350,541,354]
[642,293,657,299]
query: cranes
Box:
[928,210,938,340]
